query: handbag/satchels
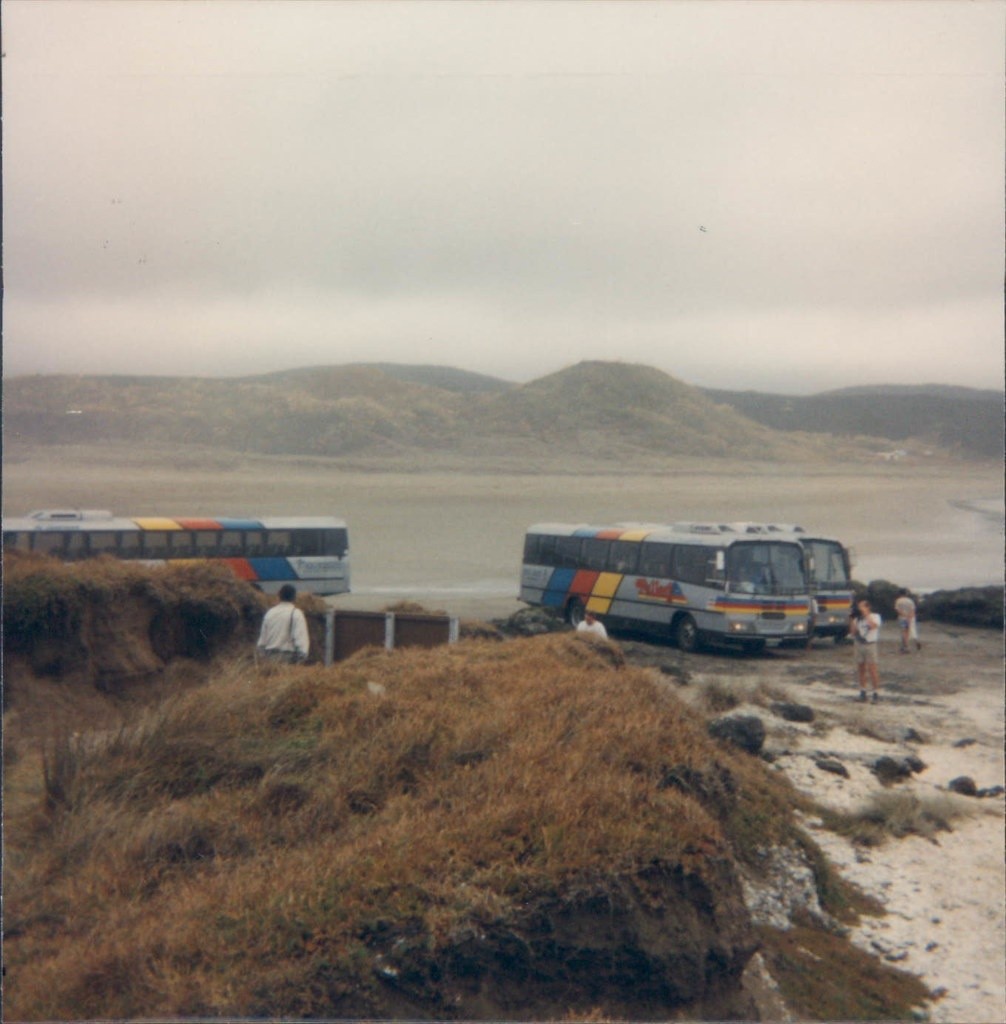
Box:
[287,650,306,665]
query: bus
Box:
[613,522,856,648]
[517,522,813,652]
[0,509,352,598]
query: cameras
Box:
[850,609,861,618]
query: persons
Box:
[576,613,607,641]
[255,584,310,665]
[894,589,922,653]
[845,600,881,705]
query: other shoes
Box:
[859,690,867,702]
[871,692,879,705]
